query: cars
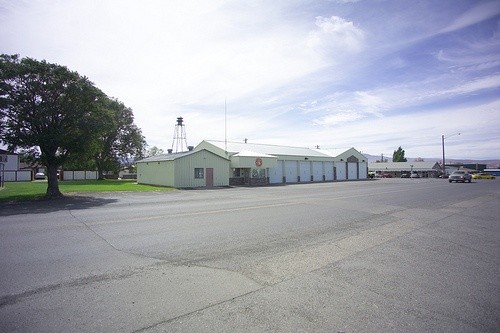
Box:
[368,171,450,179]
[448,170,472,183]
[35,173,44,179]
[471,172,496,179]
